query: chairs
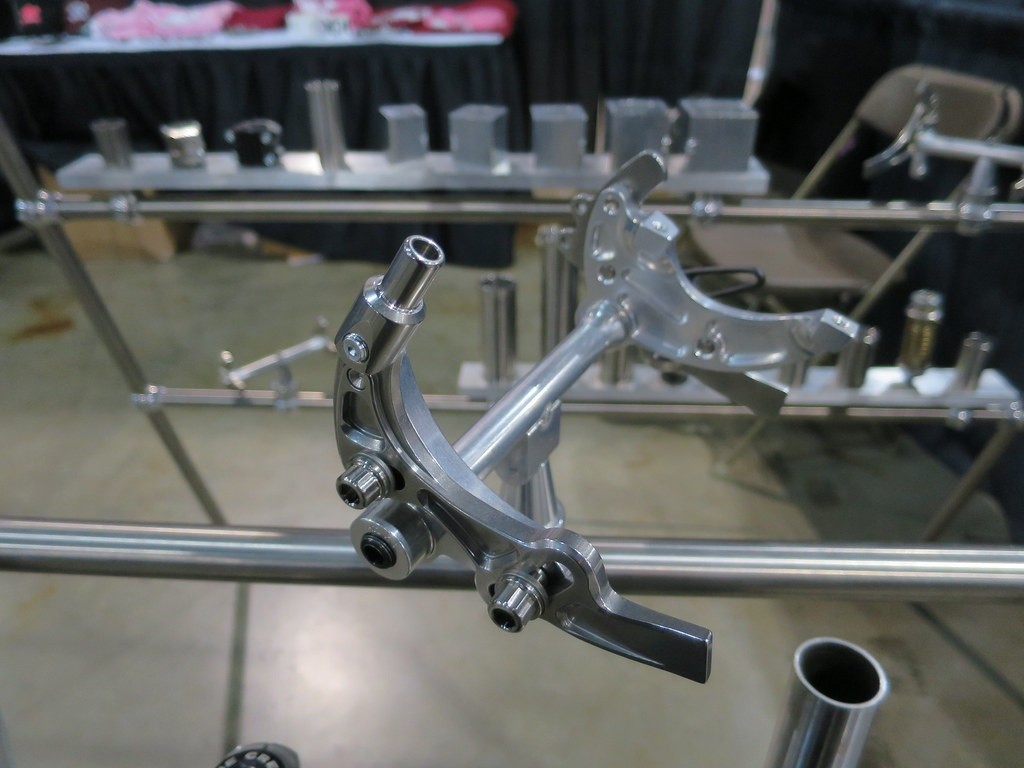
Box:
[665,64,1022,473]
